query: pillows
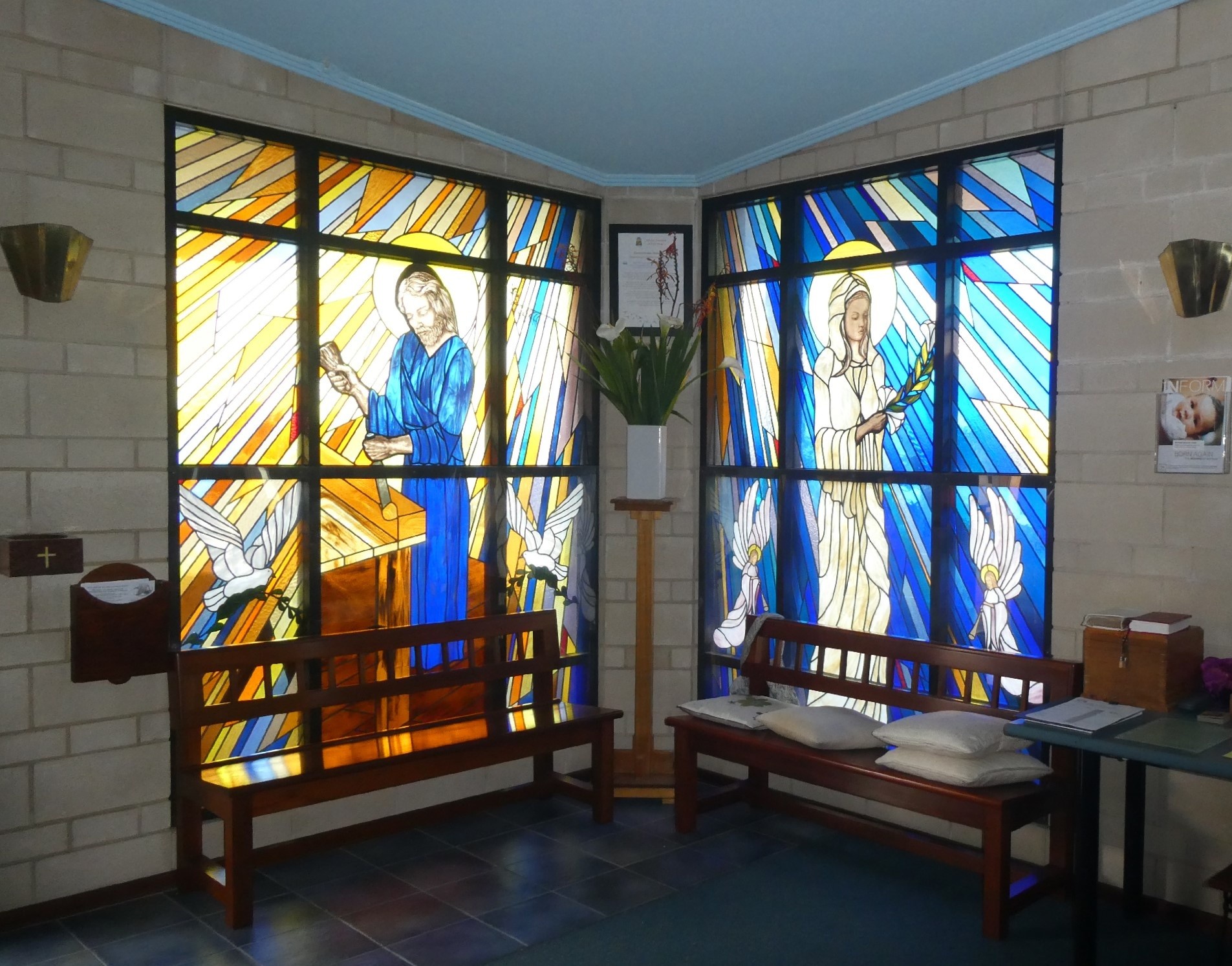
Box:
[755,705,890,752]
[875,751,1054,790]
[675,692,800,735]
[874,711,1035,760]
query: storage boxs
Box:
[1081,625,1204,711]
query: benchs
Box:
[167,603,625,928]
[661,610,1079,939]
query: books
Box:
[1197,710,1230,724]
[1080,607,1193,635]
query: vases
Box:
[626,423,668,498]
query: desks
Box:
[603,493,688,803]
[1004,687,1232,966]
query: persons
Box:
[1159,391,1224,445]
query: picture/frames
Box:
[607,224,694,337]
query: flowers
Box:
[559,262,746,424]
